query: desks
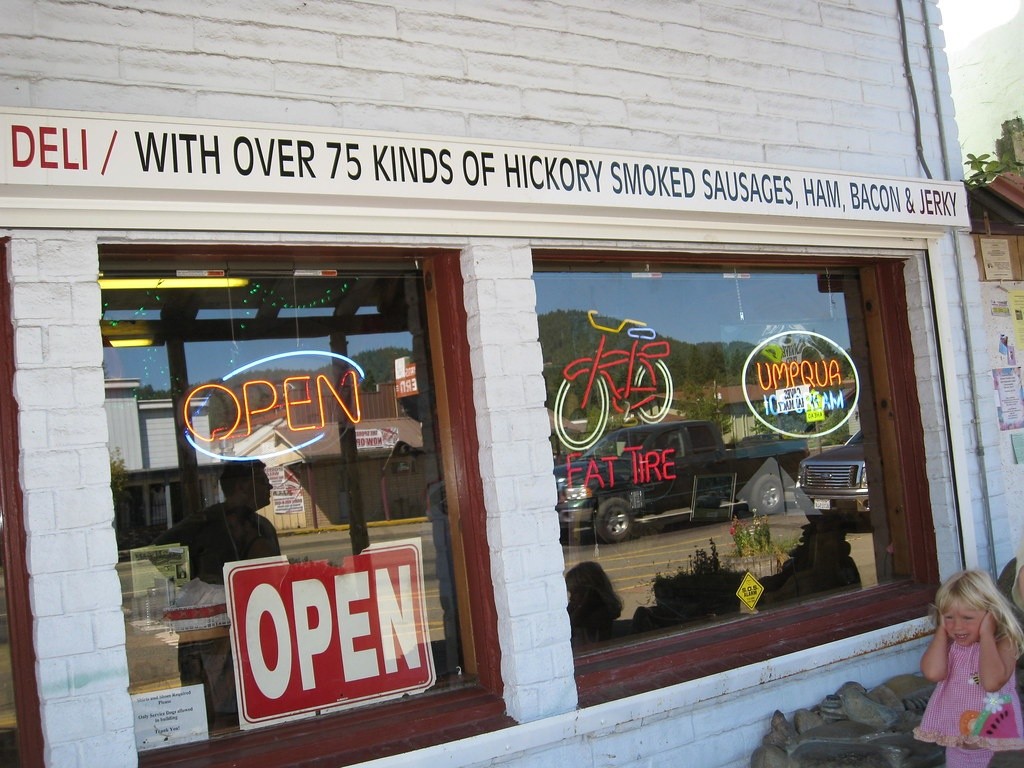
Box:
[124,616,231,724]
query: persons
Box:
[911,567,1023,768]
[225,504,278,560]
[564,562,623,649]
[1010,525,1024,611]
[143,456,282,591]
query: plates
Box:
[163,603,228,618]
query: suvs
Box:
[793,427,871,534]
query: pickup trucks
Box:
[552,419,811,545]
